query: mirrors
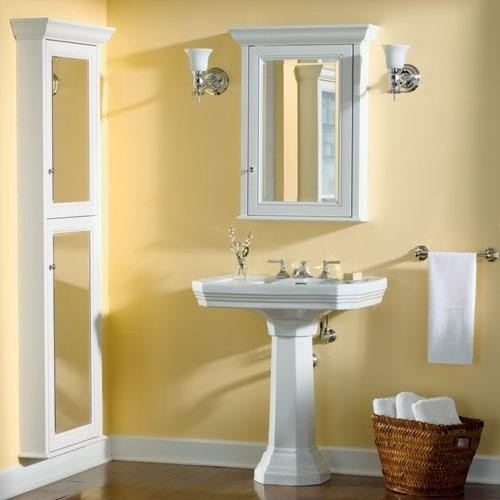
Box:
[257,55,342,208]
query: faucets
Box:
[290,259,311,279]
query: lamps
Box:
[380,43,420,102]
[184,47,230,102]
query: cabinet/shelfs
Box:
[9,12,117,460]
[226,23,381,225]
[294,62,338,205]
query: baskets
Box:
[371,414,484,498]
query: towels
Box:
[371,389,462,429]
[423,249,479,370]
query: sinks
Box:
[191,272,388,320]
